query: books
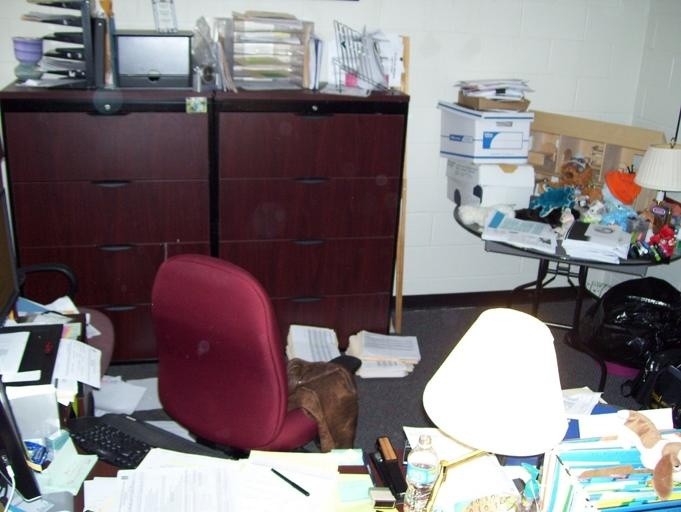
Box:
[540,430,681,511]
[285,325,422,379]
[0,378,61,497]
[480,211,632,265]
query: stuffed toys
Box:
[540,149,602,207]
[602,409,681,500]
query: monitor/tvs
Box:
[0,382,45,502]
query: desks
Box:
[452,206,681,393]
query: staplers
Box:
[369,436,408,503]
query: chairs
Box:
[151,253,362,451]
[0,186,115,386]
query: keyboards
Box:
[68,411,229,470]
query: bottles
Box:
[402,434,442,512]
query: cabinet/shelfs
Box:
[213,88,409,349]
[1,85,215,365]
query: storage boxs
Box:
[437,100,535,164]
[446,160,534,208]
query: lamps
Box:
[634,143,681,247]
[421,308,570,511]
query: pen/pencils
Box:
[270,468,310,497]
[631,240,670,264]
